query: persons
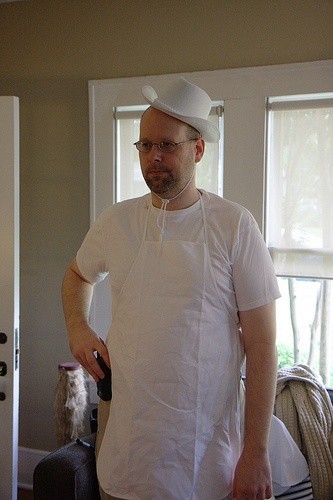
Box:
[62,75,282,500]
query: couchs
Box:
[33,377,333,500]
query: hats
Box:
[141,77,221,145]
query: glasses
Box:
[134,138,196,153]
